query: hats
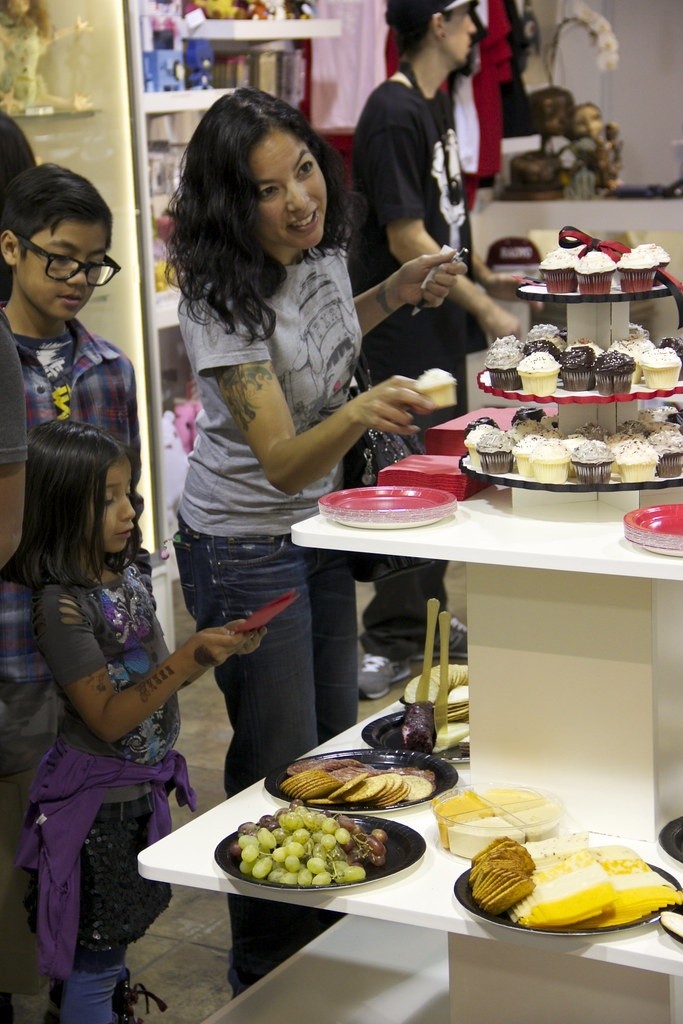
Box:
[386,0,469,31]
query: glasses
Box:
[17,236,120,286]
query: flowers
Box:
[544,1,618,88]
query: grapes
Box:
[226,798,388,887]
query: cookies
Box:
[467,836,535,915]
[280,769,434,808]
[404,662,469,706]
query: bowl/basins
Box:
[432,785,567,860]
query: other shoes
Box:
[48,970,167,1024]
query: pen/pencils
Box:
[412,248,468,316]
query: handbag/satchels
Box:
[347,348,438,582]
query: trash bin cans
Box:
[486,237,543,342]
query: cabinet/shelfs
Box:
[0,0,683,1024]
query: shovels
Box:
[434,611,450,732]
[416,598,440,702]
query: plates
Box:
[624,503,683,558]
[453,862,683,936]
[317,486,458,530]
[214,814,426,892]
[659,815,683,863]
[658,908,683,945]
[265,748,459,813]
[361,711,470,764]
[399,695,414,706]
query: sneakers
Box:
[410,615,467,660]
[358,653,410,699]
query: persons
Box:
[168,89,466,1003]
[0,114,265,1024]
[0,0,90,112]
[353,0,540,699]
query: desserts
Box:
[538,242,670,296]
[414,367,457,408]
[482,322,683,396]
[464,405,683,484]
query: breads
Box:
[401,701,436,755]
